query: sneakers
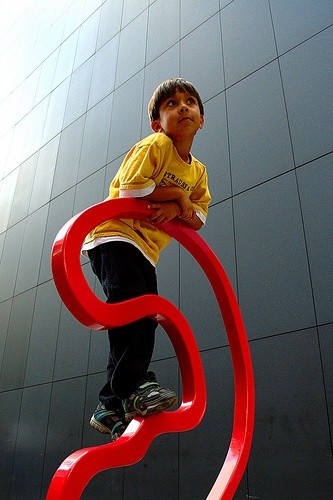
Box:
[89,401,130,442]
[122,381,177,421]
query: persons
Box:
[80,77,212,442]
